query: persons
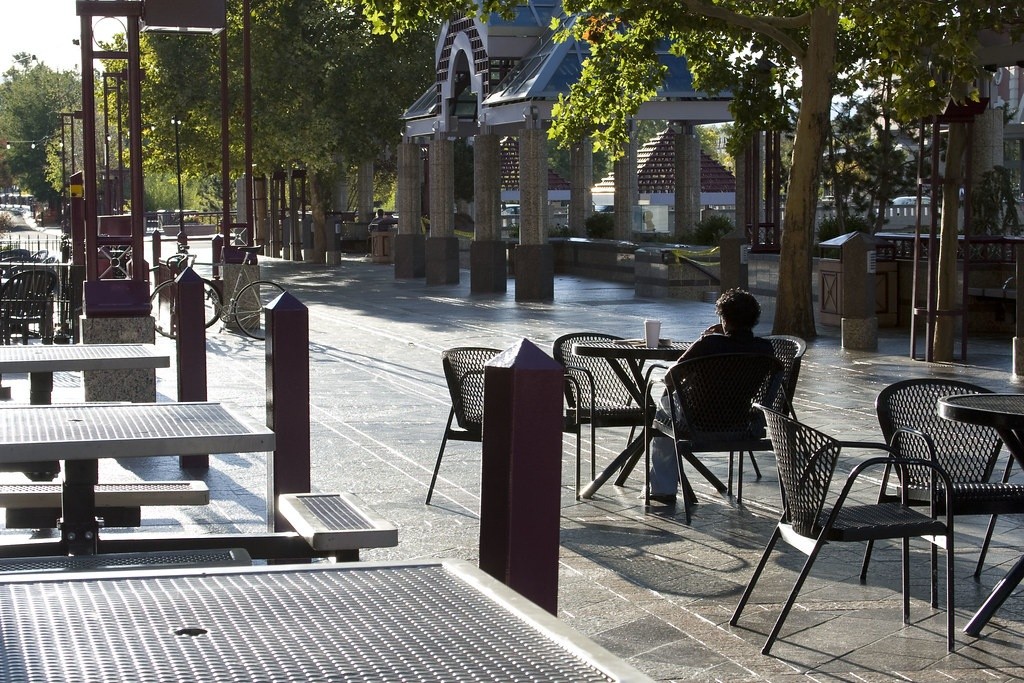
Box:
[649,287,774,503]
[368,209,384,233]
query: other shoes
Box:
[648,493,678,505]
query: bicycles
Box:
[149,244,289,341]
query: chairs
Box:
[645,352,793,523]
[42,257,55,272]
[728,402,955,655]
[0,269,57,345]
[552,332,646,481]
[858,379,1017,585]
[4,249,49,279]
[424,347,582,506]
[727,334,821,489]
[0,249,30,274]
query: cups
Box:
[644,319,662,347]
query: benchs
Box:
[0,548,252,575]
[277,490,398,563]
[0,480,210,528]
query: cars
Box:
[873,194,942,218]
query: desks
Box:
[0,558,655,683]
[571,339,727,498]
[0,342,171,482]
[0,400,299,566]
[937,392,1024,638]
[0,278,54,345]
